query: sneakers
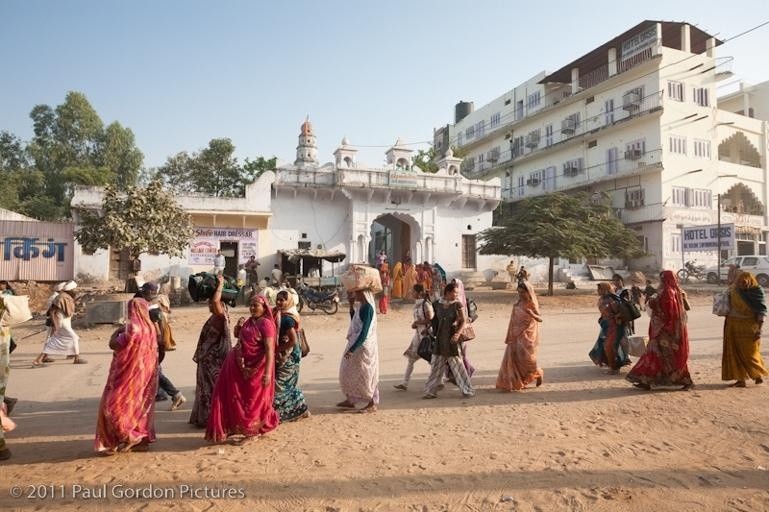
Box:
[168,395,186,410]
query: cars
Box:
[706,255,769,288]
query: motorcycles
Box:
[280,280,341,317]
[678,259,709,284]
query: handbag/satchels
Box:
[447,297,478,341]
[418,333,435,361]
[162,318,177,351]
[609,293,641,321]
[301,329,310,357]
[713,285,731,315]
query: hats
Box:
[138,282,158,291]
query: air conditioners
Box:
[461,93,643,209]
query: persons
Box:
[495,280,545,393]
[514,265,529,286]
[2,248,475,460]
[506,260,518,284]
[588,263,768,393]
